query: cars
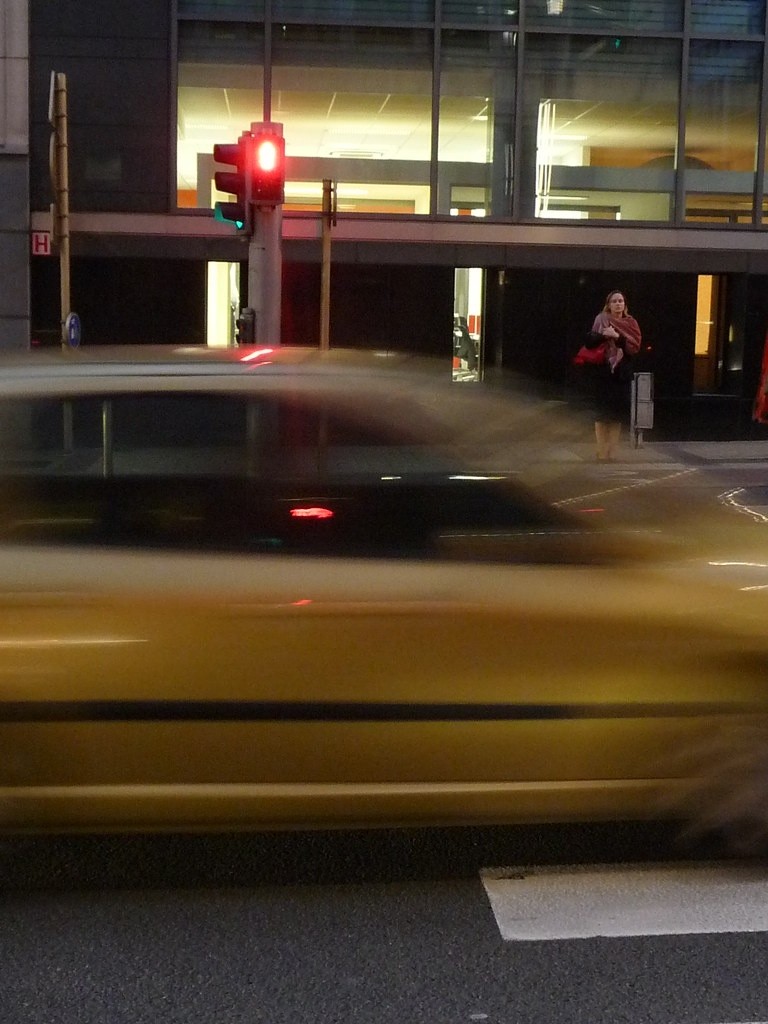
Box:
[0,347,768,864]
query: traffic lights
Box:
[234,317,250,345]
[252,134,285,201]
[214,145,245,230]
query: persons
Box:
[591,290,642,463]
